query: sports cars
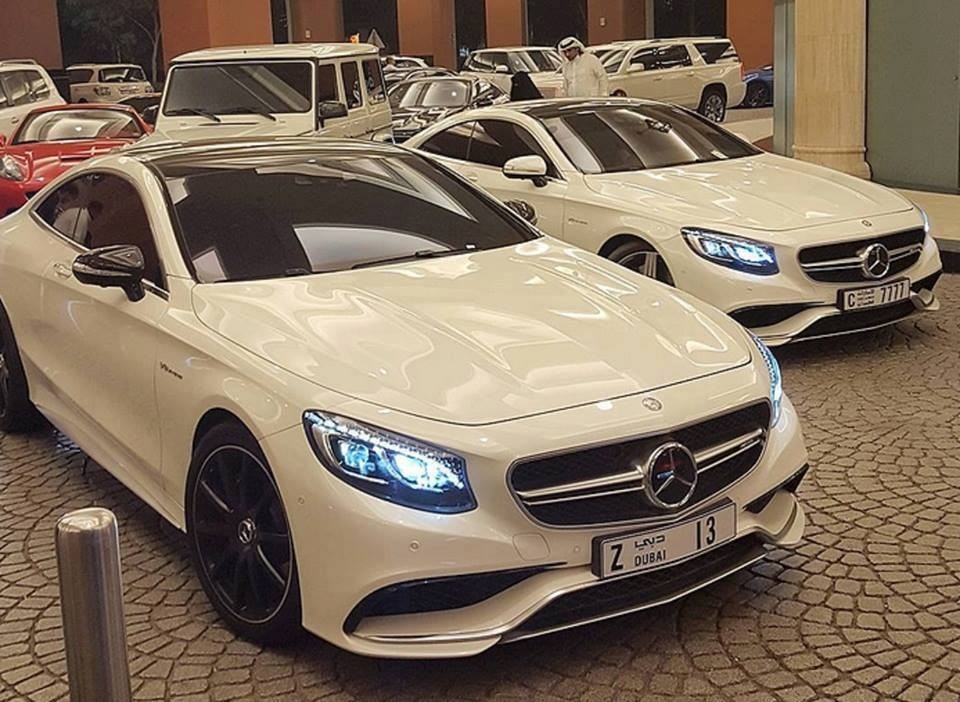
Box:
[0,103,155,219]
[397,95,943,347]
[0,134,812,661]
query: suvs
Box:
[108,42,396,179]
[533,36,746,124]
[460,46,565,99]
[0,58,65,141]
[65,63,153,103]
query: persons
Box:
[381,56,399,70]
[558,36,611,97]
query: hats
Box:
[560,39,580,51]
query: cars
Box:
[384,65,460,89]
[380,56,428,71]
[727,64,773,108]
[386,74,510,143]
